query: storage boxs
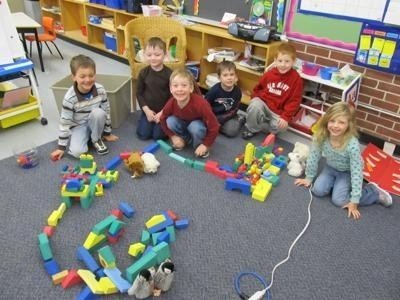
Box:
[53,75,133,129]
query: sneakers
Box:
[369,180,393,208]
[243,129,258,140]
[93,139,110,155]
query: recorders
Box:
[228,21,276,44]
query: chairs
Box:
[124,16,188,111]
[24,16,63,62]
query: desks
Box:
[10,11,44,72]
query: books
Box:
[207,47,264,72]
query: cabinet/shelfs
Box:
[40,0,282,107]
[287,65,362,141]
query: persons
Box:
[52,54,119,158]
[203,61,246,137]
[241,43,304,139]
[136,37,173,140]
[160,69,219,158]
[294,101,393,219]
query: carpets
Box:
[0,109,400,300]
